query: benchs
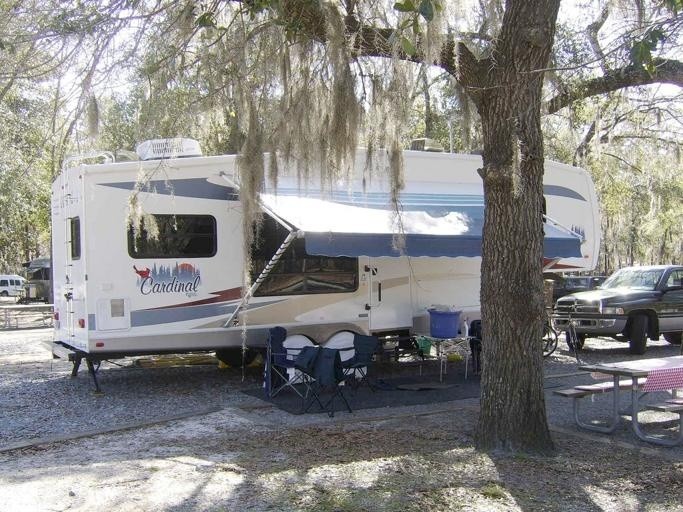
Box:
[553,378,683,447]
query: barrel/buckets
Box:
[412,315,431,335]
[427,308,462,338]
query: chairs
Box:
[266,326,378,418]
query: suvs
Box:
[553,265,682,355]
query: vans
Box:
[1,275,30,297]
[24,268,52,303]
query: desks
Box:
[419,334,475,383]
[573,355,683,446]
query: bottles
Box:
[460,319,469,338]
